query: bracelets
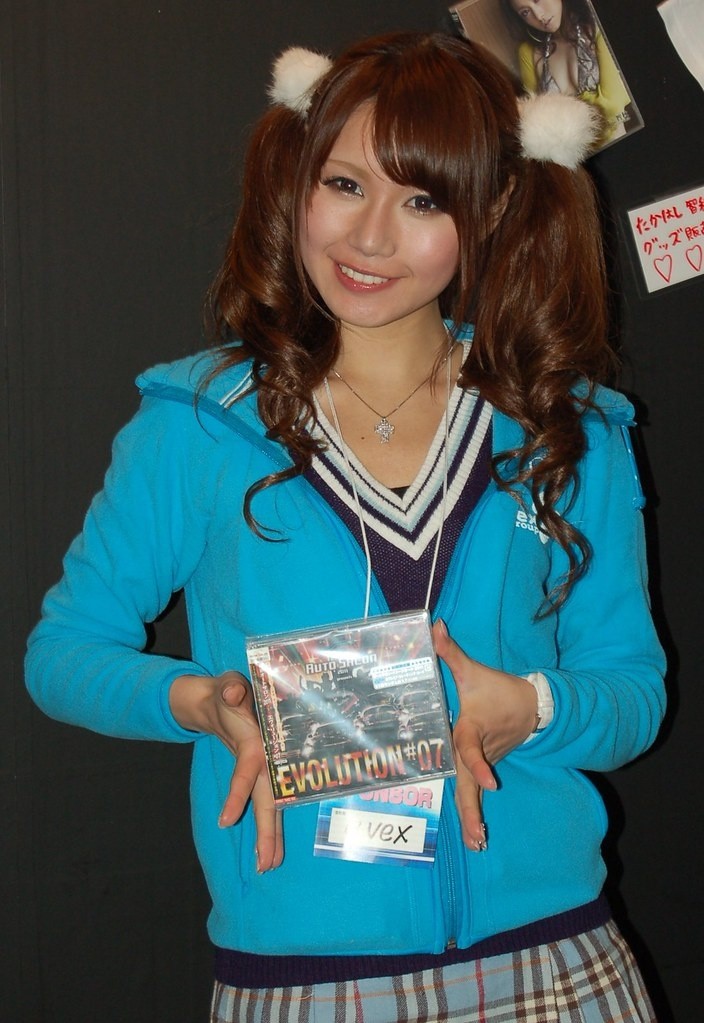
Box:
[520,669,555,748]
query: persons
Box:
[19,26,669,1022]
[505,0,635,156]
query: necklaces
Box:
[323,313,458,441]
